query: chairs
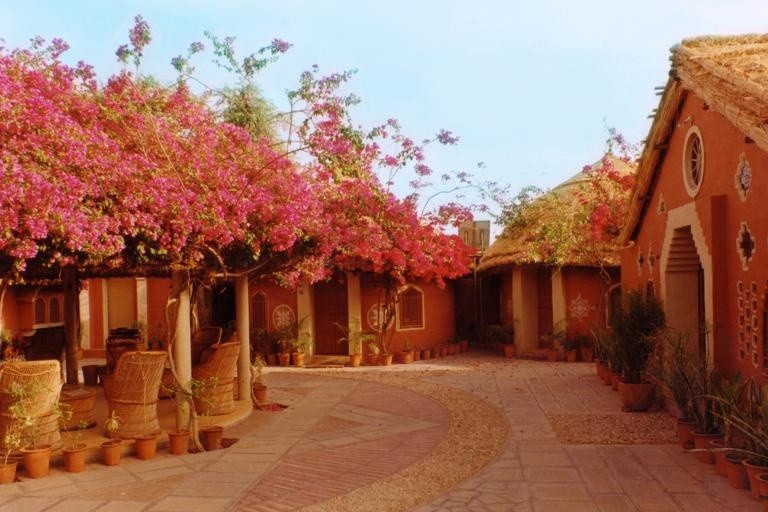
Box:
[1,359,65,449]
[99,349,171,439]
[190,327,242,417]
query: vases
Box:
[413,341,467,360]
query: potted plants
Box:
[500,334,516,357]
[537,285,667,410]
[252,314,311,369]
[643,320,768,512]
[332,316,412,368]
[1,379,225,485]
[250,355,269,404]
